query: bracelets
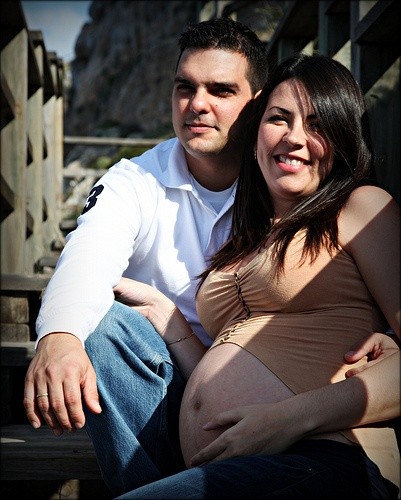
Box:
[167,332,196,346]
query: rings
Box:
[35,393,49,397]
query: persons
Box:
[114,54,401,500]
[23,18,401,495]
